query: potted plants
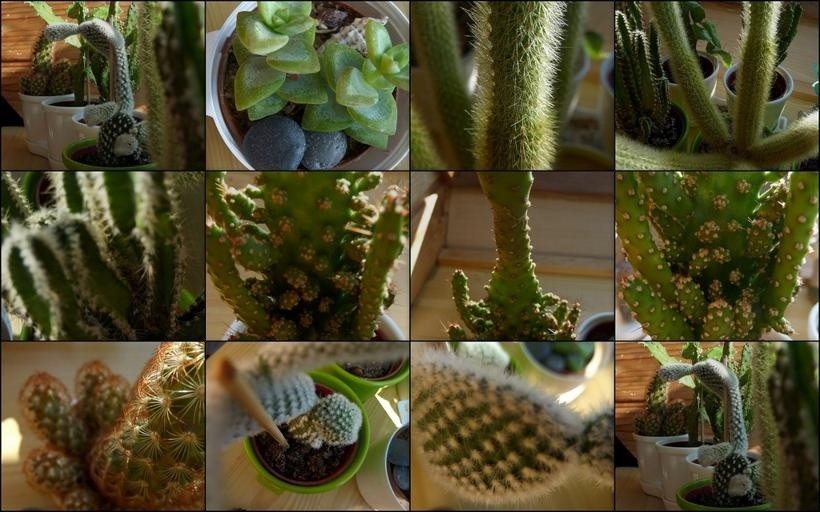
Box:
[206,171,410,342]
[506,342,604,405]
[207,343,410,512]
[18,19,159,169]
[632,360,772,511]
